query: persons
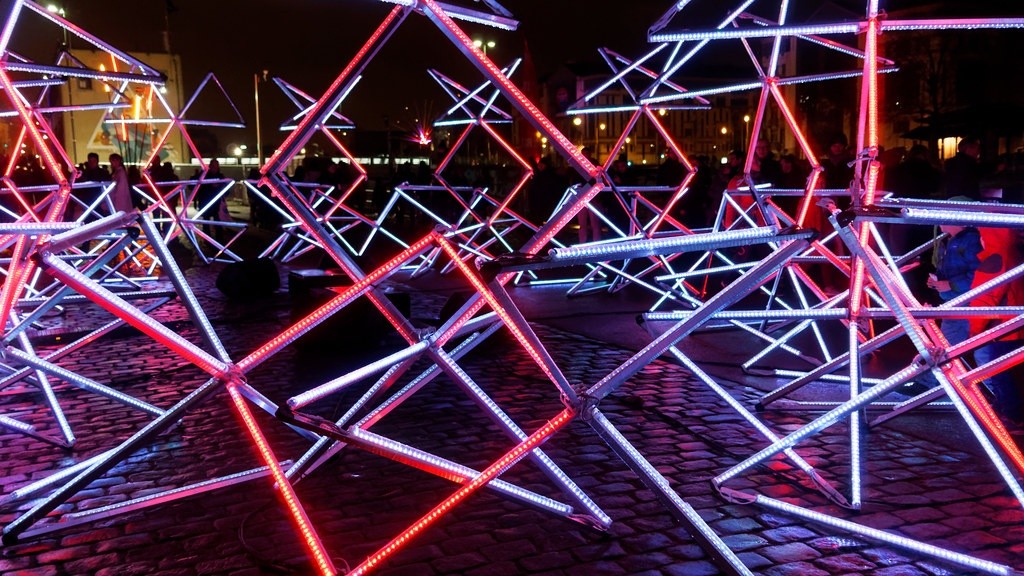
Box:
[895,196,983,399]
[1,135,1024,262]
[967,225,1024,396]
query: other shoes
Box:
[894,382,929,396]
